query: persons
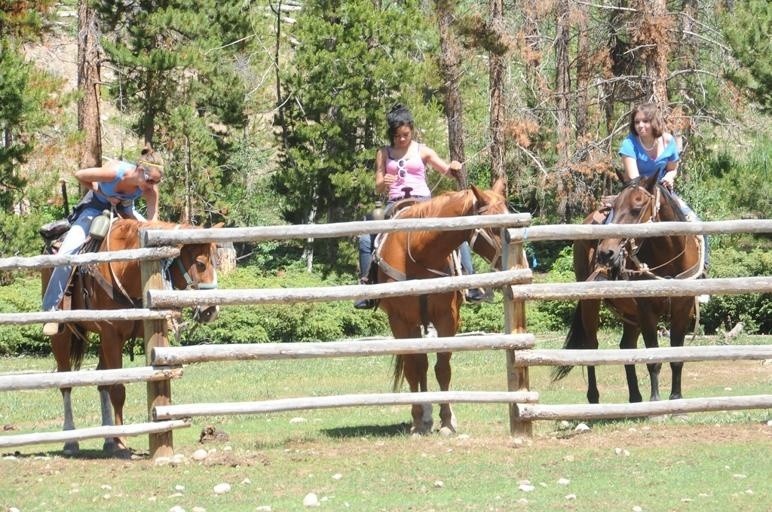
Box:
[41,146,165,335]
[354,104,484,309]
[618,103,710,304]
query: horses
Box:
[547,167,704,402]
[372,175,535,438]
[37,215,226,457]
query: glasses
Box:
[145,172,162,185]
[398,160,408,180]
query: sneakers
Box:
[466,288,484,302]
[354,278,376,309]
[42,323,59,336]
